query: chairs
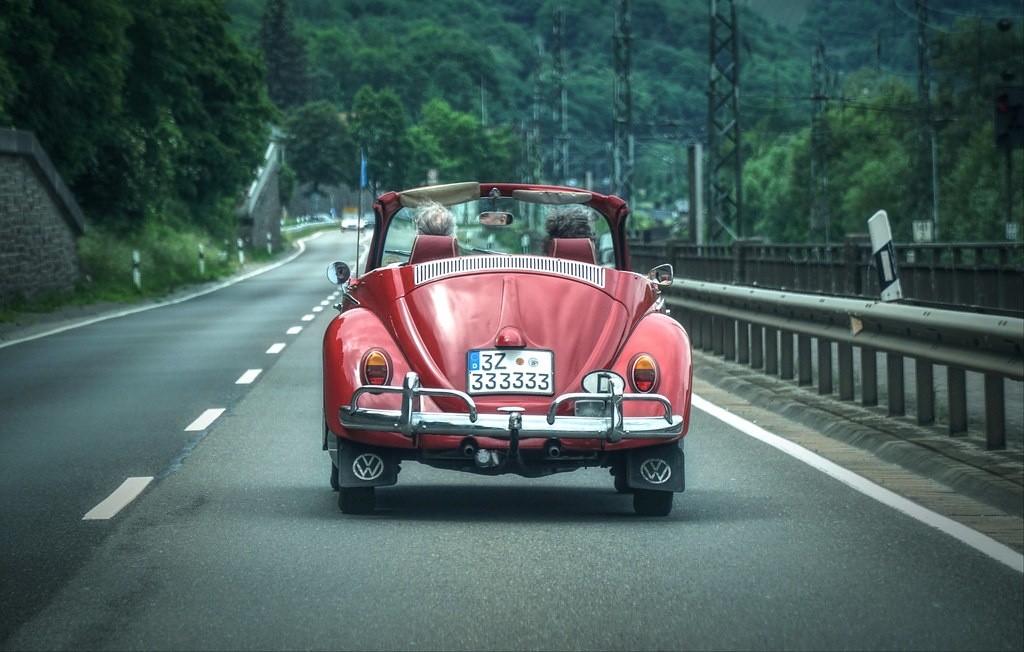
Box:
[550,238,597,266]
[407,235,458,266]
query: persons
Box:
[546,207,600,263]
[481,214,508,226]
[413,198,456,235]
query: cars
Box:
[322,181,694,517]
[340,209,375,229]
